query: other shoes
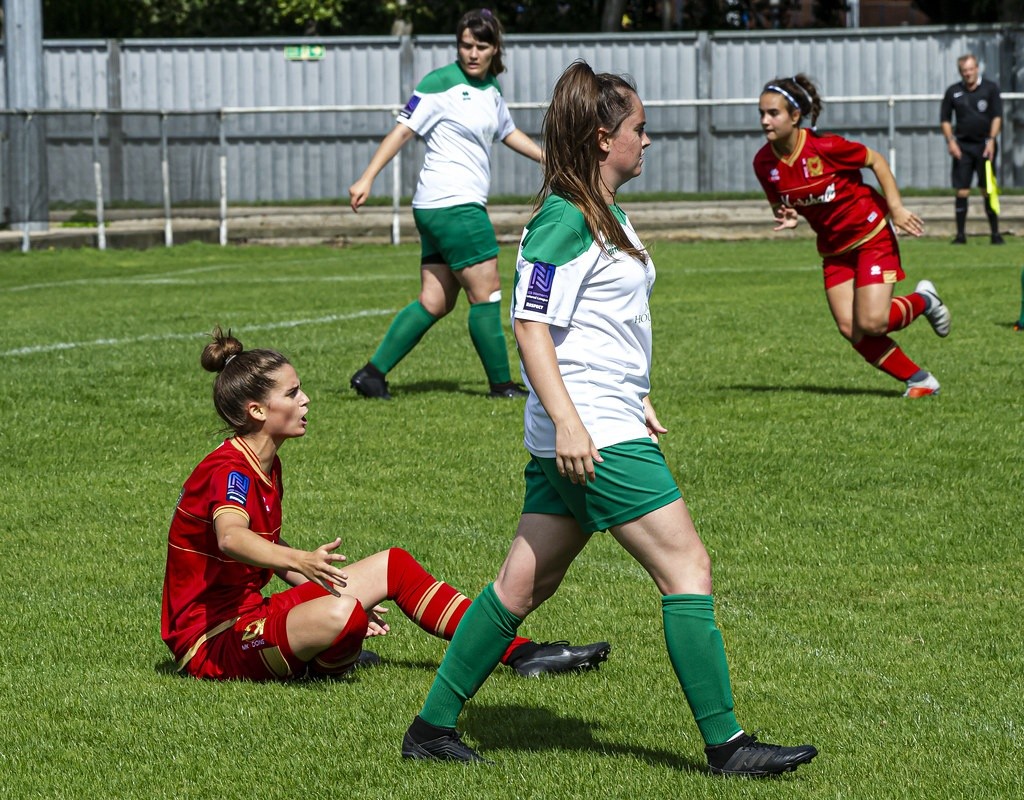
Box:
[1012,320,1024,332]
[951,235,966,244]
[992,233,1003,244]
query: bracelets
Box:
[987,135,996,139]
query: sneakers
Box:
[915,279,951,338]
[902,372,941,399]
[351,369,391,401]
[706,733,818,779]
[402,715,495,767]
[486,382,529,398]
[512,639,611,680]
[359,650,384,667]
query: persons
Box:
[940,54,1006,244]
[160,324,612,680]
[348,7,548,400]
[401,57,817,779]
[753,72,953,399]
